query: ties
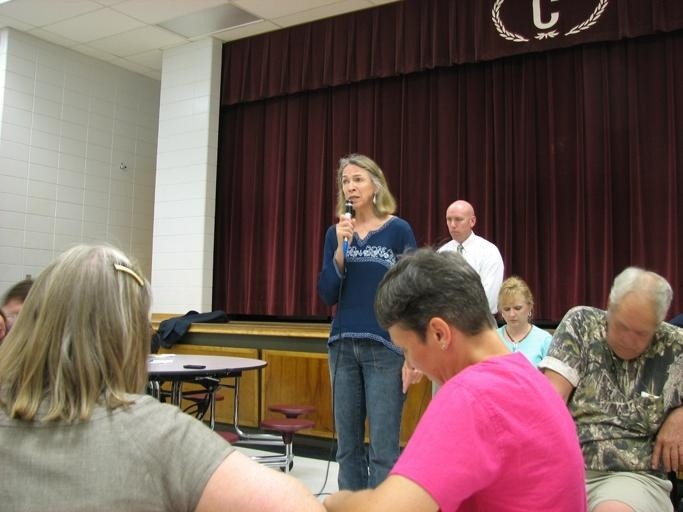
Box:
[457,245,462,255]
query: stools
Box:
[145,388,317,476]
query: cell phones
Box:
[183,364,206,368]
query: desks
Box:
[146,353,295,476]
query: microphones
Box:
[342,199,354,256]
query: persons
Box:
[433,200,505,316]
[538,266,682,512]
[496,276,553,370]
[317,153,424,491]
[323,245,587,512]
